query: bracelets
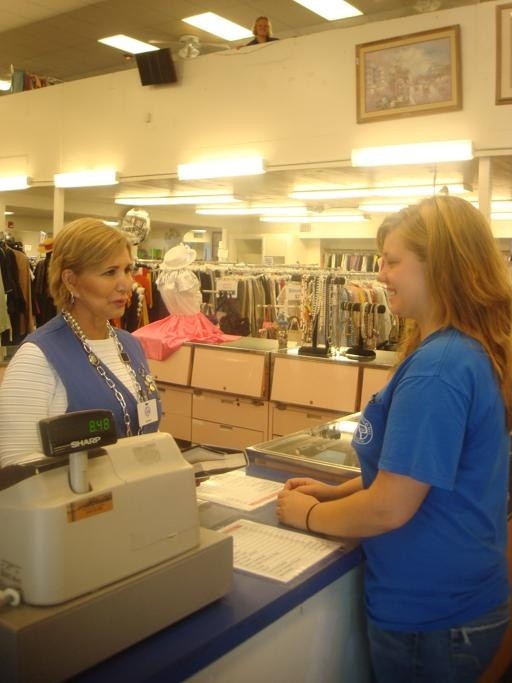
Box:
[304,502,320,532]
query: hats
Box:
[160,246,197,271]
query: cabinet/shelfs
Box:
[138,338,400,453]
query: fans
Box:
[149,35,228,50]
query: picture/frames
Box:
[355,25,463,123]
[495,3,512,105]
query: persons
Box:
[246,13,279,46]
[2,214,163,490]
[273,192,512,681]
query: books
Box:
[182,442,250,481]
[196,469,288,512]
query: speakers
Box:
[137,48,177,86]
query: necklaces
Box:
[288,269,385,355]
[61,308,148,439]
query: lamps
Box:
[261,214,369,222]
[351,141,474,168]
[0,176,33,192]
[195,204,319,216]
[113,194,244,206]
[292,183,474,200]
[53,169,119,190]
[177,157,269,180]
[179,43,199,58]
